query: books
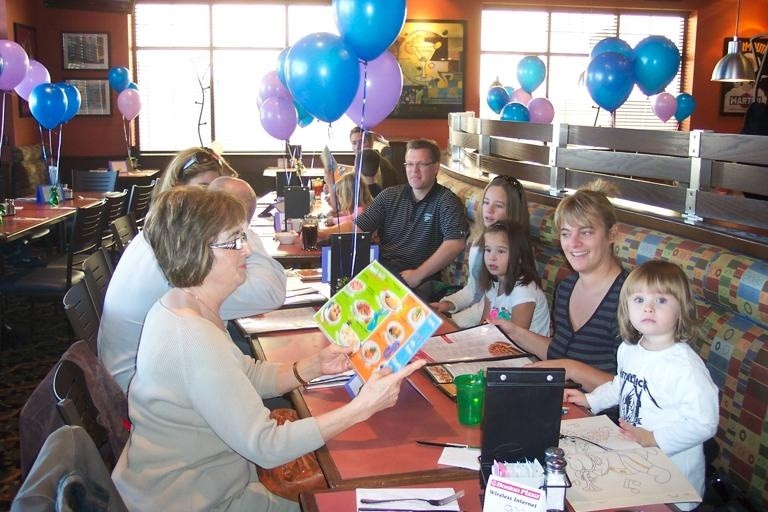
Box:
[417,321,545,401]
[553,414,704,511]
[311,257,442,390]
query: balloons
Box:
[254,1,413,142]
[1,37,81,131]
[127,82,140,91]
[486,30,695,126]
[115,88,138,121]
[108,66,132,92]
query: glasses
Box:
[181,151,218,170]
[403,161,434,167]
[208,232,248,250]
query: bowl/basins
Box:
[274,232,299,245]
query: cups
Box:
[309,178,325,207]
[451,374,484,424]
[301,222,319,251]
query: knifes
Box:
[416,439,482,451]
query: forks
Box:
[360,489,465,505]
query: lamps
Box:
[709,1,758,83]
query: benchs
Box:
[428,166,765,510]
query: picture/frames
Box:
[720,37,767,117]
[385,18,465,119]
[65,77,112,117]
[60,31,111,71]
[14,22,39,118]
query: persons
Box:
[561,257,721,511]
[93,125,406,415]
[484,188,641,429]
[94,145,291,417]
[425,173,530,329]
[295,137,468,289]
[478,218,552,338]
[110,186,429,512]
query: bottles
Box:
[4,198,16,214]
[543,444,568,511]
[49,183,73,205]
